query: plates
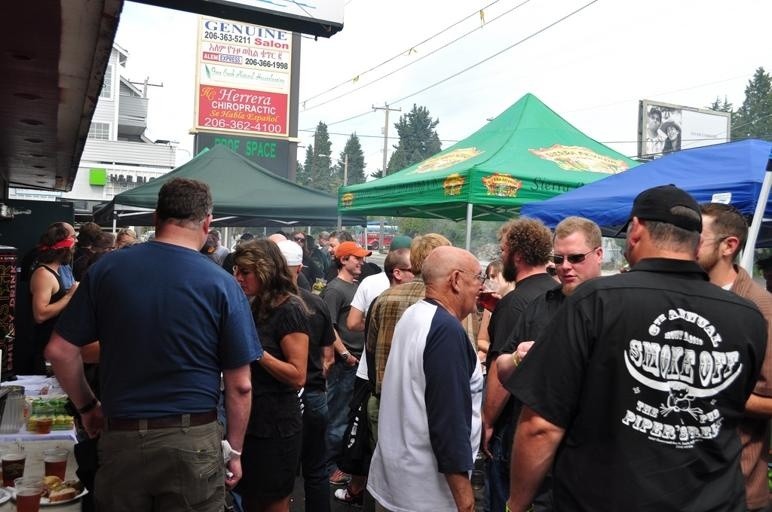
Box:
[39,487,89,505]
[0,488,12,504]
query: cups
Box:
[0,386,33,435]
[44,447,72,481]
[35,408,54,433]
[2,449,27,485]
[45,362,53,378]
[14,475,44,512]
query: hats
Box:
[661,118,682,133]
[332,241,372,260]
[277,240,303,267]
[647,108,662,118]
[389,235,412,250]
[615,184,702,239]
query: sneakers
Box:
[333,488,363,507]
[329,467,353,486]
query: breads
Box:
[48,486,77,501]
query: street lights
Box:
[317,151,350,187]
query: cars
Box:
[601,237,621,268]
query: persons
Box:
[645,108,668,159]
[13,177,771,511]
[660,121,681,155]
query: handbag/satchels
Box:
[336,383,377,477]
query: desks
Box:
[0,372,79,443]
[0,440,96,512]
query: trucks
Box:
[358,232,399,251]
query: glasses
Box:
[451,269,488,284]
[547,248,596,264]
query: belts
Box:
[104,412,217,432]
